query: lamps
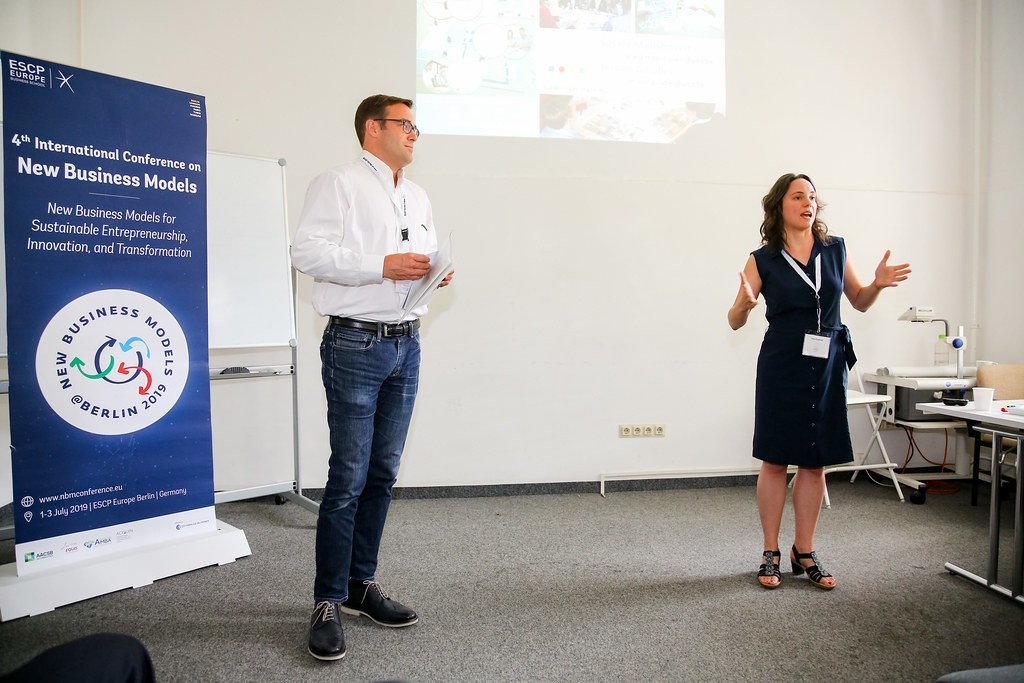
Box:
[898,307,949,338]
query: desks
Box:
[915,400,1024,607]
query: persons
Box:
[289,95,454,660]
[727,172,912,589]
[540,0,580,29]
[434,65,448,86]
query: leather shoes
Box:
[341,577,419,628]
[308,601,346,661]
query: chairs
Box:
[788,364,905,509]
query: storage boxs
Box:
[876,384,964,421]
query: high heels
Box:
[790,545,836,589]
[758,548,782,588]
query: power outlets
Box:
[618,424,665,436]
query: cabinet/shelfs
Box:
[865,372,1012,506]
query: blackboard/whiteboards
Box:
[206,149,297,348]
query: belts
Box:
[329,316,420,337]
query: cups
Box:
[972,387,995,412]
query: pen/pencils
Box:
[421,224,427,230]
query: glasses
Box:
[373,118,420,137]
